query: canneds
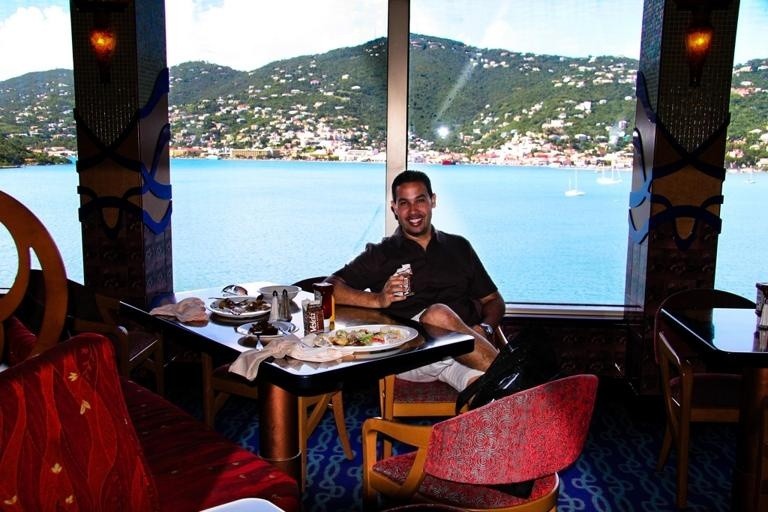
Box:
[303,303,325,336]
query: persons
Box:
[323,171,520,405]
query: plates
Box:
[206,297,272,318]
[232,320,297,338]
[329,323,420,353]
[235,336,261,349]
[208,312,270,328]
[354,334,425,359]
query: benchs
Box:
[1,310,299,511]
[0,332,157,512]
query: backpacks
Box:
[456,335,558,498]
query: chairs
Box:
[197,274,357,484]
[354,372,600,511]
[640,287,756,512]
[371,320,519,464]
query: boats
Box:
[745,178,755,183]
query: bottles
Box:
[269,291,281,321]
[280,289,292,321]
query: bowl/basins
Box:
[289,299,302,314]
[257,284,302,302]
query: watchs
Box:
[479,322,496,344]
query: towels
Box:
[225,334,360,381]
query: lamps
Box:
[72,0,128,89]
[671,0,731,87]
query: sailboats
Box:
[595,147,622,186]
[561,155,585,200]
